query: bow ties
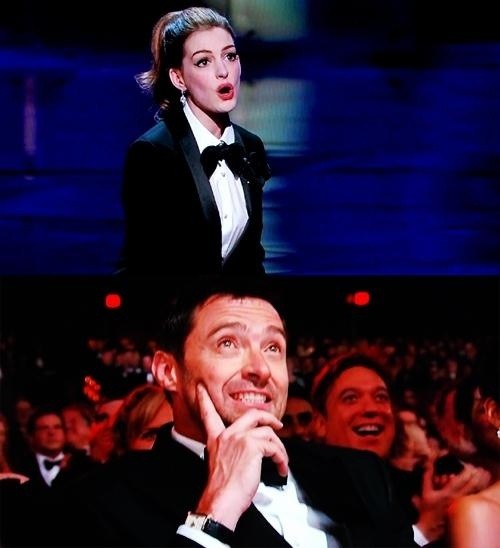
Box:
[203,447,287,486]
[200,142,244,179]
[44,458,64,471]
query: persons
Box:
[122,7,276,275]
[0,281,500,547]
